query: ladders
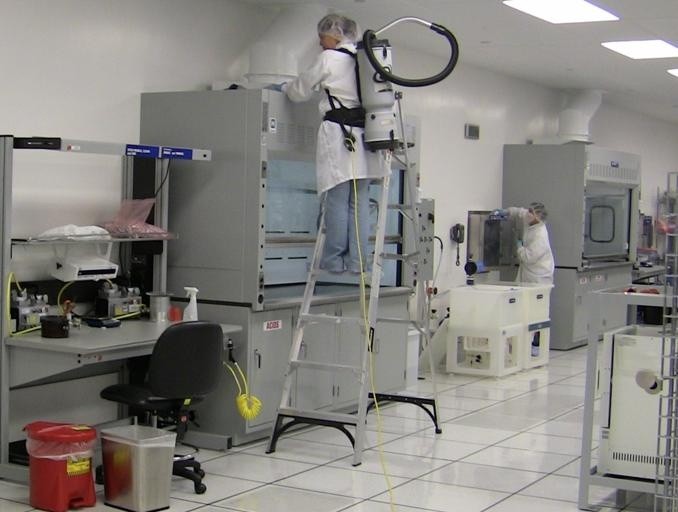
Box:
[263,90,443,467]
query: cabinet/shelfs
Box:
[338,290,413,419]
[576,281,678,512]
[550,266,636,353]
[172,303,338,448]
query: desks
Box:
[1,308,246,485]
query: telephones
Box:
[450,223,465,244]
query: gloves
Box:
[494,207,505,218]
[512,238,523,252]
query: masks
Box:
[526,213,535,226]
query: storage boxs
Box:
[472,283,556,319]
[446,287,527,322]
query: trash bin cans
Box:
[100,425,178,512]
[21,421,97,512]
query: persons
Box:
[488,204,554,283]
[264,11,393,276]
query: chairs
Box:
[94,320,224,493]
